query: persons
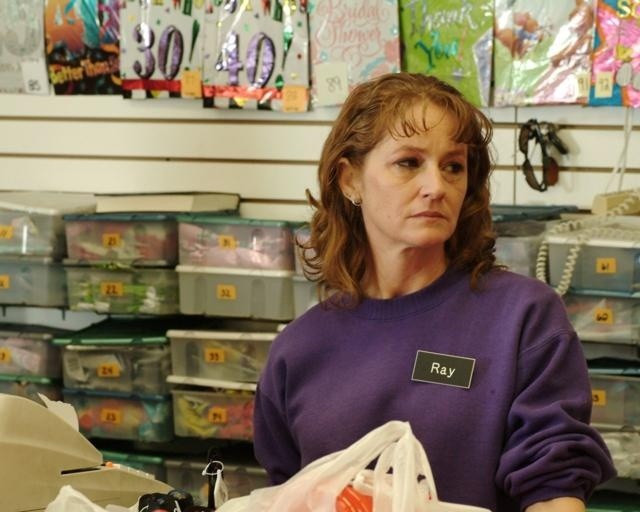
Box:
[253,72,616,512]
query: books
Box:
[93,192,242,215]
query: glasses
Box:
[536,122,559,186]
[519,119,546,192]
